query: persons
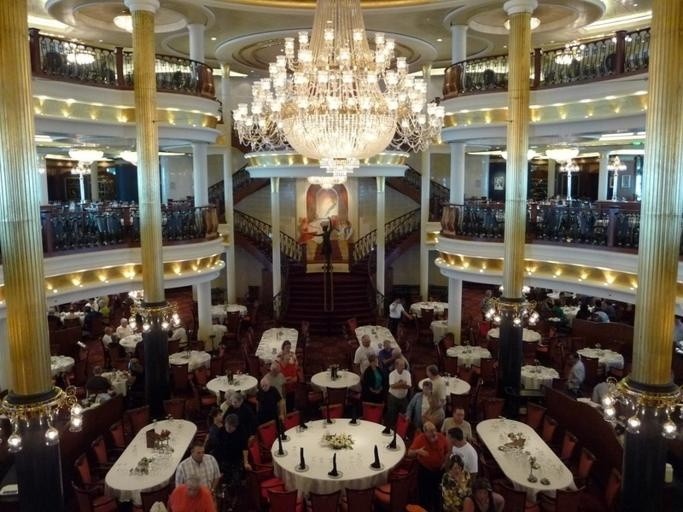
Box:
[44,287,504,511]
[529,288,617,404]
[343,220,354,242]
[298,214,316,244]
[312,216,334,265]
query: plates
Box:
[275,419,401,478]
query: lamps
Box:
[500,148,536,161]
[545,146,580,165]
[67,147,104,166]
[558,162,580,173]
[232,0,446,185]
[68,51,95,66]
[607,156,627,171]
[120,148,138,168]
[504,17,541,32]
[554,40,586,65]
[113,9,133,35]
